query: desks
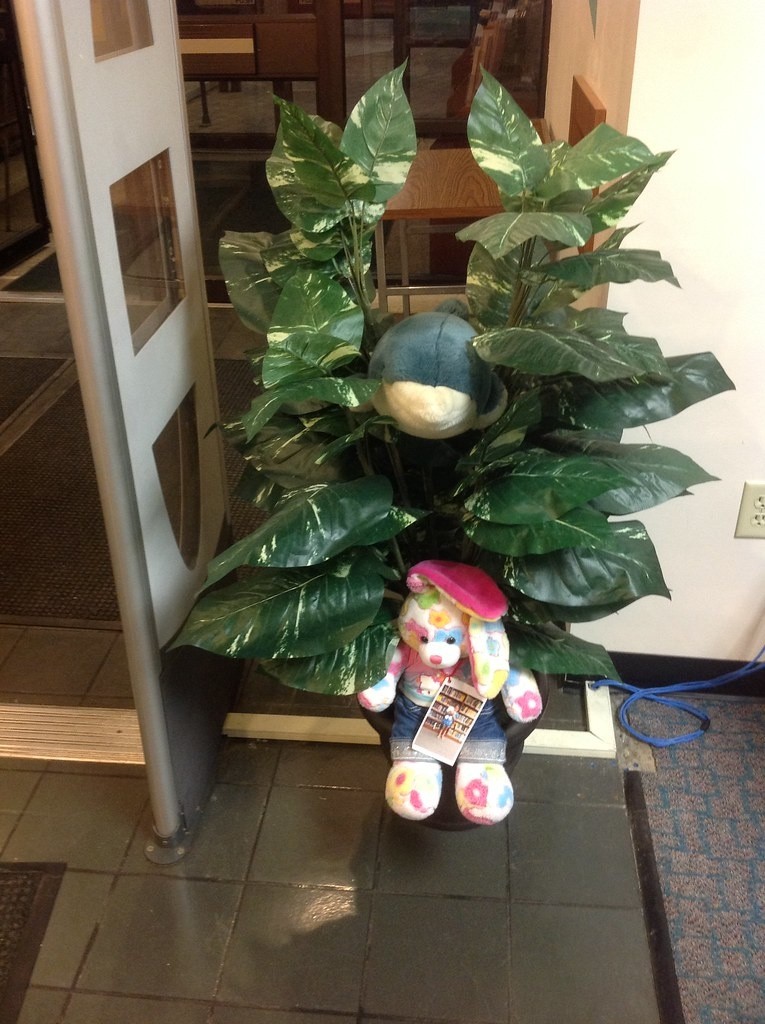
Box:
[362,149,505,319]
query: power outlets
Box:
[736,479,765,541]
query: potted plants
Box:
[165,51,734,834]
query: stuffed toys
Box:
[363,298,513,438]
[353,560,543,827]
